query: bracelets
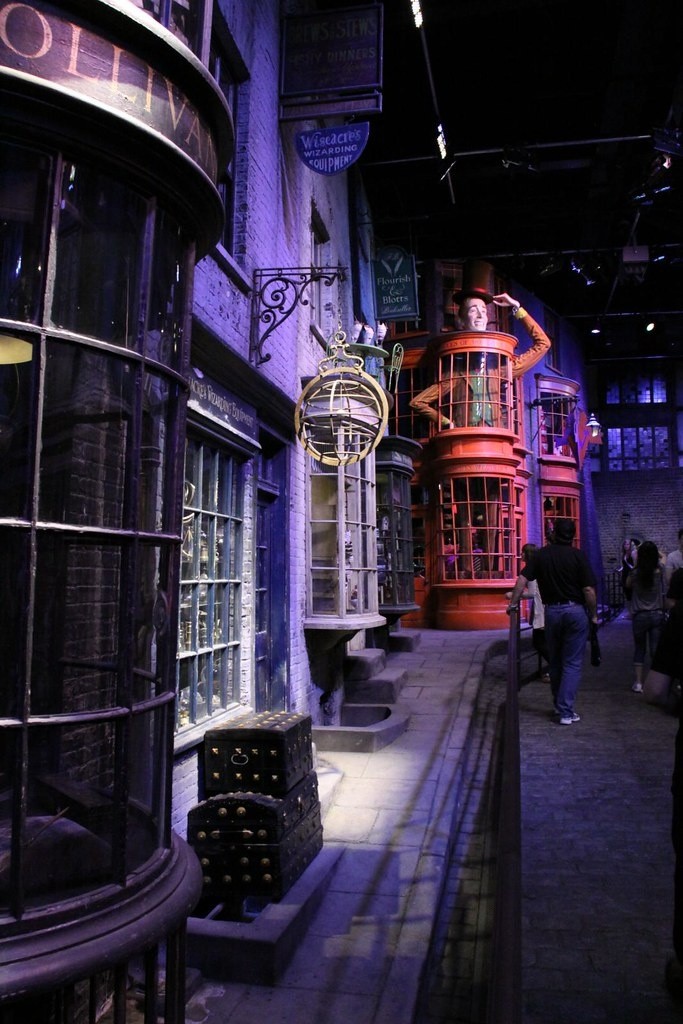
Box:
[509,601,518,608]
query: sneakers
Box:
[552,702,580,725]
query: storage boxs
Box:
[183,710,323,902]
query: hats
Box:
[552,517,577,541]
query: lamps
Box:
[584,411,600,437]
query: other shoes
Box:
[632,680,643,693]
[542,672,551,682]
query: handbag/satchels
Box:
[665,612,669,623]
[590,622,602,667]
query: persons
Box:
[644,595,683,1006]
[412,282,551,579]
[616,528,683,692]
[504,517,600,725]
